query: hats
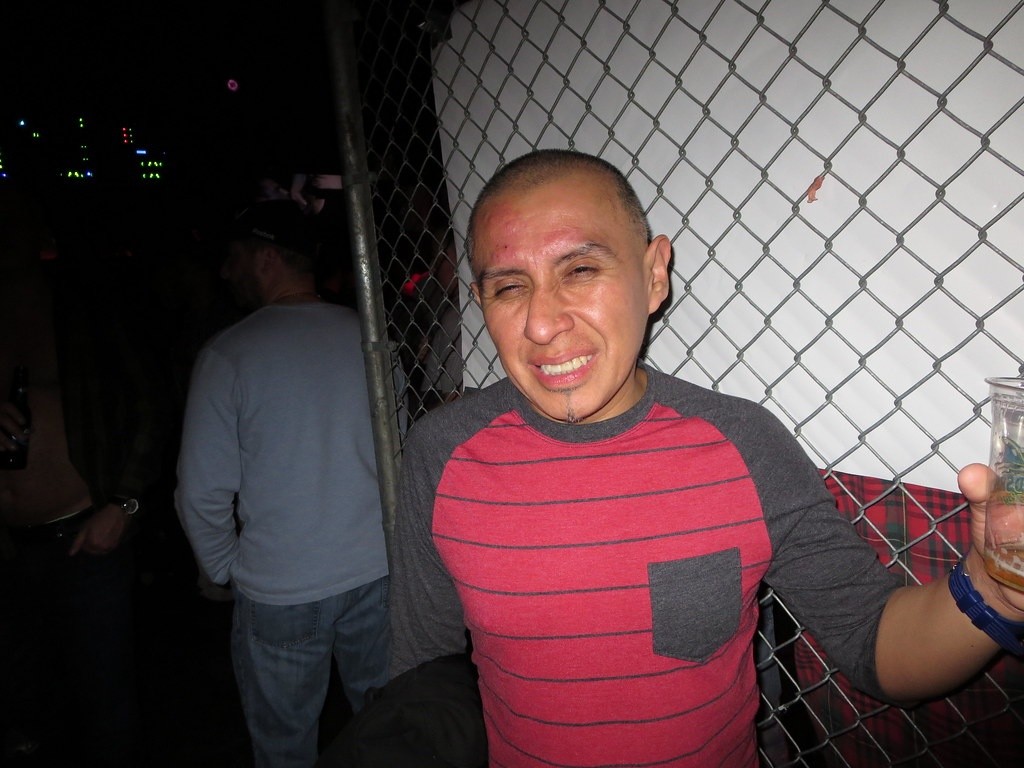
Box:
[230,195,325,260]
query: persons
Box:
[242,166,462,429]
[391,148,1024,768]
[174,197,408,767]
[0,113,165,768]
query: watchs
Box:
[108,496,139,514]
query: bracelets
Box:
[947,556,1024,656]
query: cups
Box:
[982,377,1024,592]
